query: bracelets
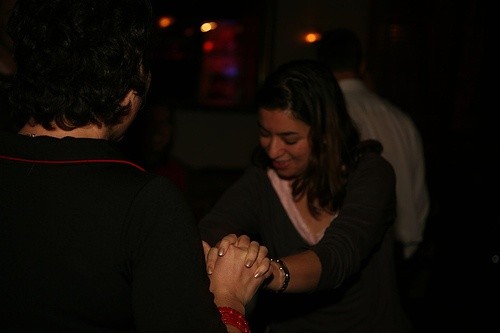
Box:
[217,307,251,333]
[270,257,290,294]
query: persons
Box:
[196,61,412,333]
[0,0,274,333]
[328,46,429,318]
